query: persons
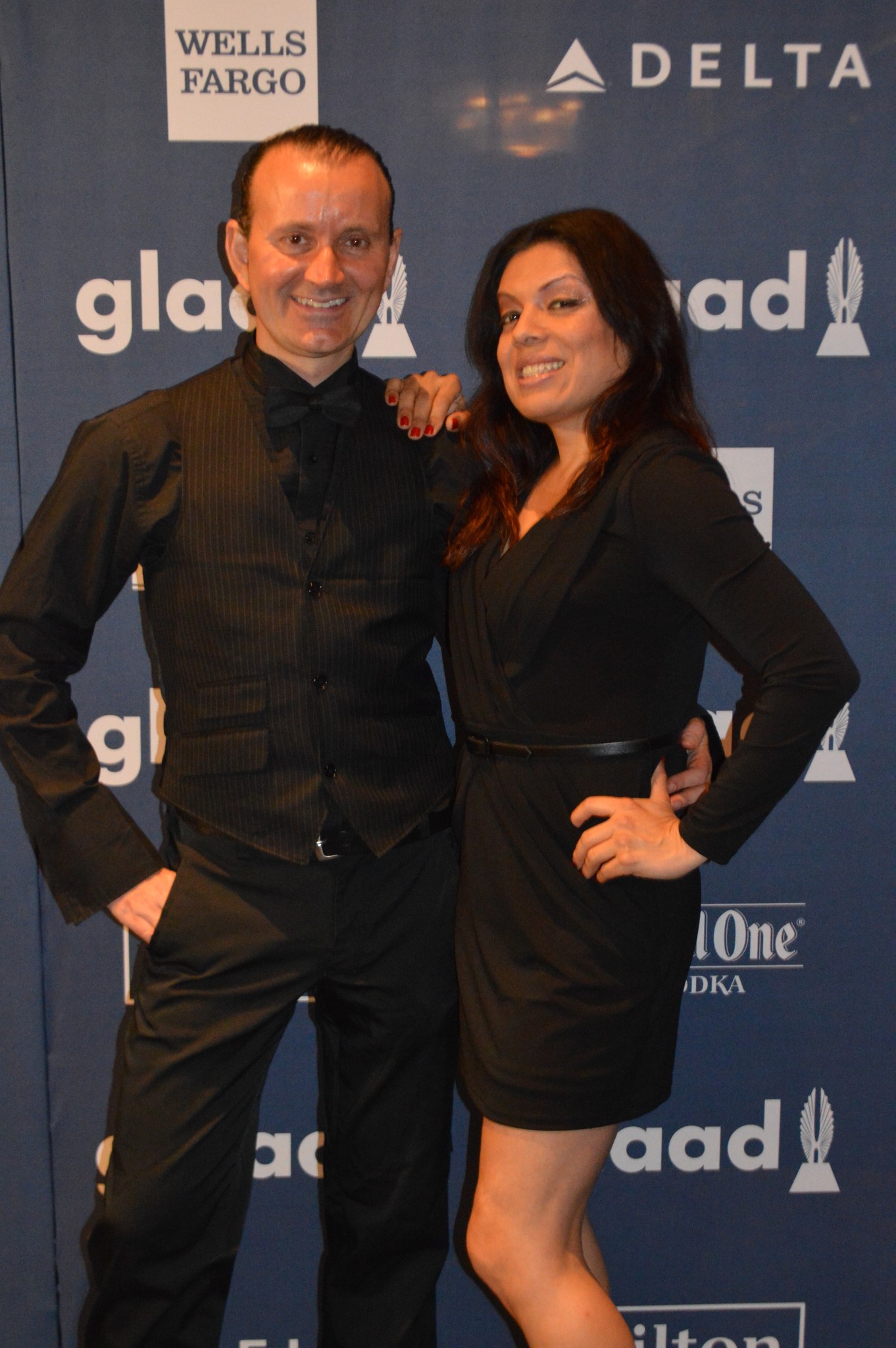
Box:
[379,200,864,1345]
[4,123,733,1348]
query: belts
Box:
[316,804,455,870]
[461,733,680,762]
